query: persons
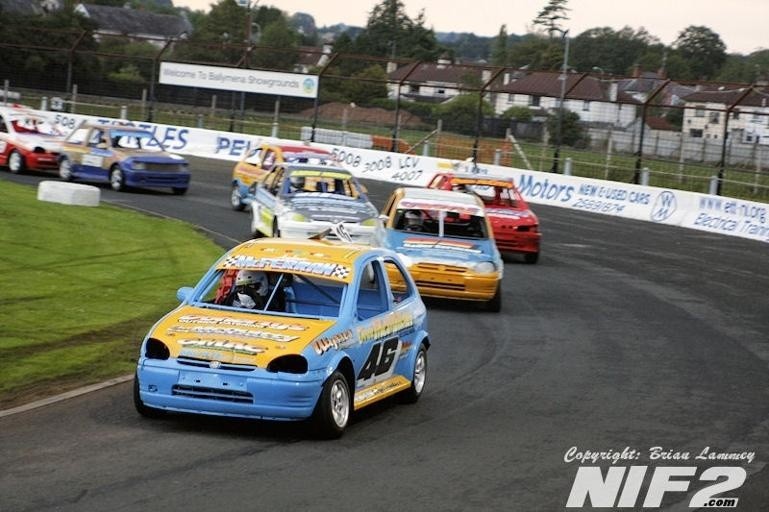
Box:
[288,174,309,193]
[233,268,285,312]
[405,210,423,233]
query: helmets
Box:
[405,211,424,227]
[289,176,305,192]
[235,269,269,309]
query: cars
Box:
[426,173,540,264]
[248,163,380,243]
[229,146,332,212]
[56,124,191,195]
[281,154,368,197]
[133,236,430,436]
[372,188,504,312]
[0,107,70,174]
